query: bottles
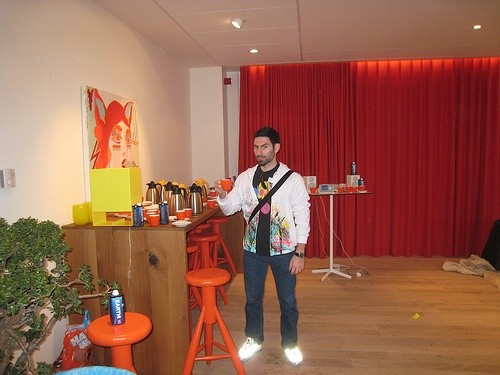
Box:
[160,201,169,225]
[359,178,364,186]
[352,162,356,175]
[109,289,125,325]
[134,203,144,227]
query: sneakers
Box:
[239,337,262,361]
[284,345,303,366]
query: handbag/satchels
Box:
[50,310,95,373]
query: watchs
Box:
[295,252,305,257]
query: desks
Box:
[309,191,372,282]
[61,198,245,375]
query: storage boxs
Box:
[303,176,317,192]
[89,167,143,227]
[347,175,360,187]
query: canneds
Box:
[132,203,144,227]
[158,201,170,225]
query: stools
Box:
[185,216,246,375]
[87,311,152,375]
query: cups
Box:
[310,187,317,194]
[176,210,185,220]
[141,201,160,227]
[207,200,216,208]
[338,186,358,193]
[359,186,365,191]
[183,209,192,218]
[220,179,232,191]
[73,202,93,225]
[210,190,216,197]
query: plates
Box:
[172,221,191,227]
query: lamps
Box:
[232,19,244,28]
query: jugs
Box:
[164,182,173,203]
[195,177,208,202]
[145,181,163,204]
[169,184,186,216]
[189,182,204,216]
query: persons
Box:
[214,126,310,366]
